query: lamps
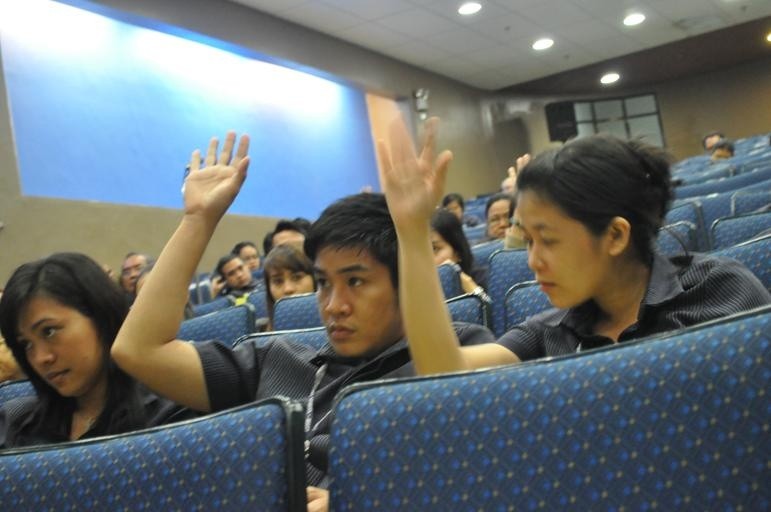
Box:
[601,74,620,84]
[458,2,482,16]
[623,13,646,27]
[532,37,554,51]
[767,32,771,42]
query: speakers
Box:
[544,101,578,142]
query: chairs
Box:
[189,295,237,317]
[328,302,771,512]
[663,200,709,252]
[436,263,463,299]
[230,325,331,351]
[444,293,485,327]
[244,287,270,316]
[503,280,554,334]
[513,281,517,282]
[0,393,308,512]
[652,219,699,255]
[704,230,770,296]
[0,377,38,407]
[159,127,769,300]
[487,246,536,343]
[710,211,771,251]
[171,305,254,347]
[730,190,771,216]
[274,292,323,330]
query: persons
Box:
[117,251,194,321]
[485,195,512,239]
[230,240,259,270]
[501,153,532,249]
[108,131,496,511]
[702,132,723,151]
[376,115,771,374]
[211,255,260,306]
[710,139,734,161]
[131,265,155,296]
[429,208,491,304]
[0,251,202,450]
[263,221,307,254]
[442,193,480,227]
[263,241,317,334]
